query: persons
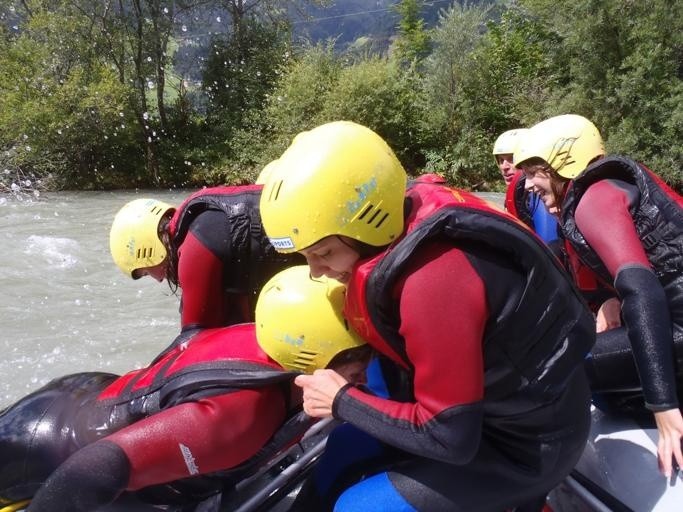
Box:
[0,263,375,512]
[491,125,560,250]
[259,120,600,512]
[509,110,681,479]
[110,183,308,329]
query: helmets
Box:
[109,198,177,280]
[492,115,606,180]
[254,120,407,375]
